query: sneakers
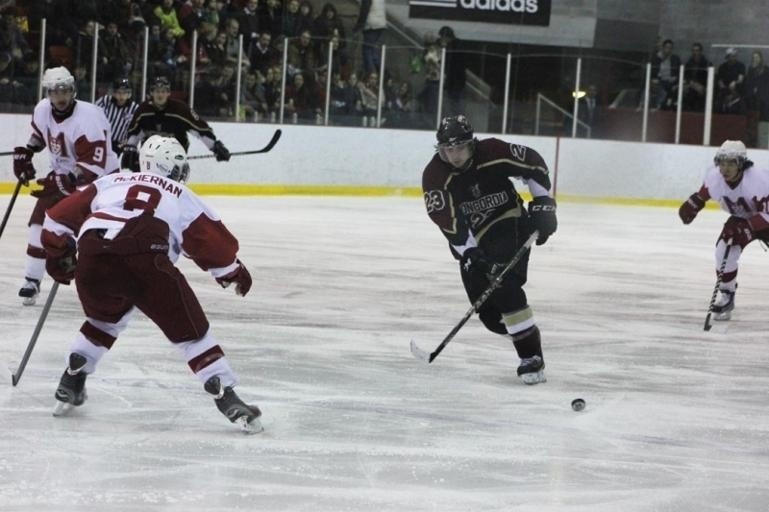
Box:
[52,369,90,407]
[708,289,736,314]
[517,352,547,377]
[213,385,266,427]
[17,275,43,298]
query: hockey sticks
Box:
[12,280,59,385]
[703,237,733,330]
[185,128,282,159]
[409,230,538,365]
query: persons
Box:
[424,114,557,384]
[564,84,598,137]
[96,80,141,168]
[40,132,262,434]
[0,0,466,129]
[677,138,769,321]
[638,37,768,122]
[124,74,231,176]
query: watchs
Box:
[14,66,117,305]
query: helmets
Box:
[713,138,748,168]
[434,114,475,145]
[146,77,172,95]
[42,66,76,90]
[137,134,188,184]
[111,78,133,91]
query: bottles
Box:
[291,111,298,124]
[362,116,376,127]
[253,110,259,123]
[271,111,276,124]
[315,109,322,125]
[229,107,233,118]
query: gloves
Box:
[11,144,38,188]
[209,139,235,164]
[120,145,139,172]
[525,195,561,244]
[216,258,256,298]
[461,247,510,288]
[44,254,77,288]
[677,192,708,225]
[30,170,76,200]
